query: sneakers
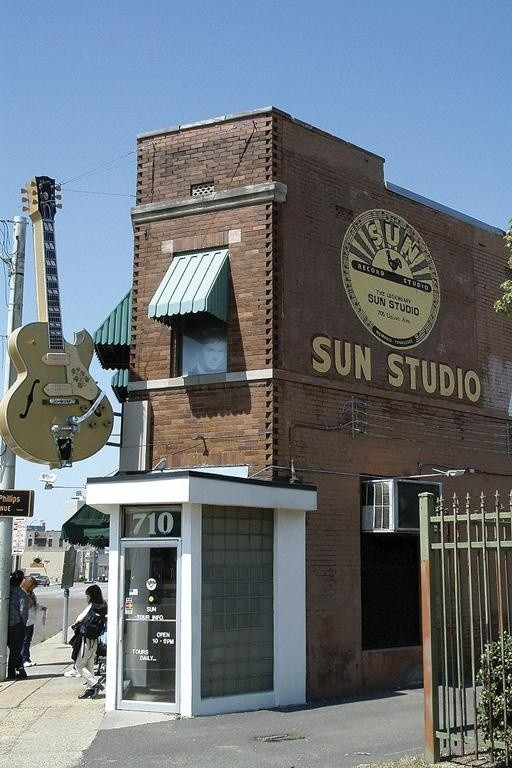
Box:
[64,668,82,678]
[8,661,36,679]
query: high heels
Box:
[78,682,105,699]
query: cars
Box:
[26,573,50,587]
[98,573,108,583]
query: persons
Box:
[11,570,30,678]
[21,576,45,668]
[73,585,108,699]
[64,621,108,677]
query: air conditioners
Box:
[361,480,443,535]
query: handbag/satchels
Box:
[79,602,107,640]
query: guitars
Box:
[0,175,113,469]
